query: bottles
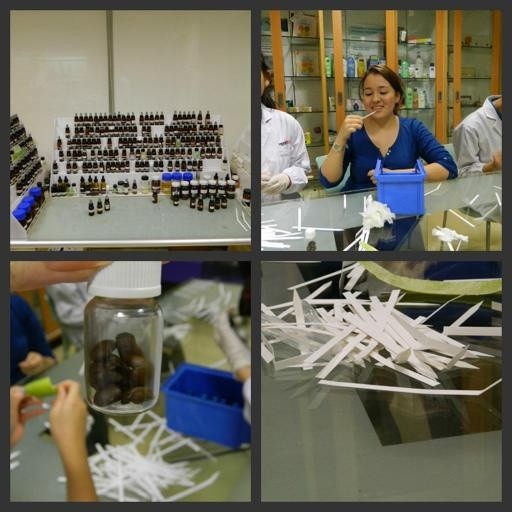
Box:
[406,87,426,108]
[243,188,251,207]
[9,114,51,230]
[399,54,435,78]
[325,53,386,77]
[84,261,163,416]
[51,109,240,216]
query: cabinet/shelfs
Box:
[262,10,502,178]
[50,117,232,195]
[10,114,49,239]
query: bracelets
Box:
[331,142,346,152]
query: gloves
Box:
[211,307,254,380]
[262,172,291,194]
[260,172,271,190]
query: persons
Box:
[451,93,501,227]
[9,290,62,387]
[8,376,99,504]
[317,61,459,256]
[260,51,313,204]
[9,261,113,296]
[46,258,252,428]
